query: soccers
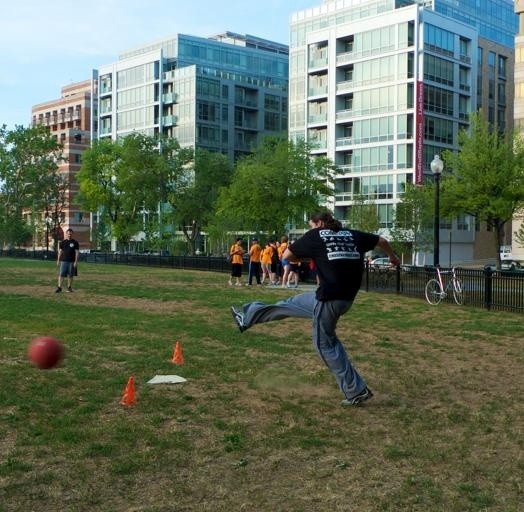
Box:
[30,337,61,368]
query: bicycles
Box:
[425,262,467,306]
[374,266,404,295]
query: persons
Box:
[227,204,402,407]
[53,228,80,294]
[227,235,301,289]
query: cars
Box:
[366,257,417,277]
[484,260,524,276]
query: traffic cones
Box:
[118,375,136,406]
[171,340,186,365]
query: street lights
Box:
[430,154,445,302]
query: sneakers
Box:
[230,305,248,332]
[339,388,373,405]
[56,287,62,293]
[68,287,74,292]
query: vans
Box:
[500,245,512,259]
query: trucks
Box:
[79,249,101,253]
[139,249,169,257]
